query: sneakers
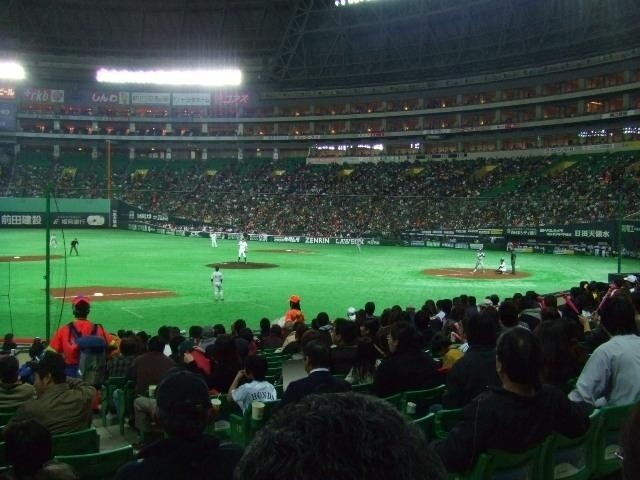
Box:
[133,442,149,448]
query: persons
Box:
[1,97,639,238]
[236,238,248,265]
[471,249,486,273]
[209,231,219,249]
[210,266,225,303]
[495,256,507,274]
[504,239,640,260]
[1,273,640,479]
[69,237,80,256]
[49,233,58,249]
[509,250,516,275]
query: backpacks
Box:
[67,321,107,386]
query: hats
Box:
[26,350,65,373]
[72,297,90,313]
[348,307,357,320]
[479,299,493,308]
[288,296,300,303]
[157,370,212,416]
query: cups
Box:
[211,398,222,410]
[148,385,156,397]
[252,401,267,421]
[406,402,417,413]
